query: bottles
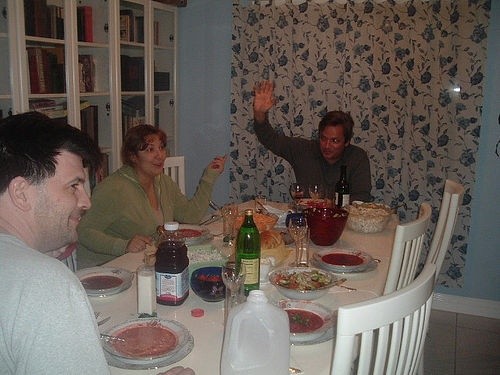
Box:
[334,164,350,208]
[155,221,189,307]
[234,208,261,296]
[220,290,290,375]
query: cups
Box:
[221,261,246,323]
[221,203,238,246]
[307,208,349,246]
[260,258,271,282]
[255,195,267,213]
[290,183,325,199]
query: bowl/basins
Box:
[268,267,334,301]
[342,201,395,234]
[190,267,239,303]
[291,199,333,210]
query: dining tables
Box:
[88,201,399,375]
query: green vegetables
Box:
[279,270,319,291]
[289,314,310,326]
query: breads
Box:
[258,230,282,249]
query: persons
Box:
[253,80,371,204]
[76,125,229,266]
[0,109,110,375]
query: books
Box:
[25,0,169,192]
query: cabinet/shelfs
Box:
[0,0,180,175]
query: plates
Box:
[152,223,214,248]
[311,248,377,274]
[71,261,336,370]
[230,210,306,265]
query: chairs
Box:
[384,202,432,295]
[425,178,464,285]
[330,263,437,375]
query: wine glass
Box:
[286,213,309,268]
